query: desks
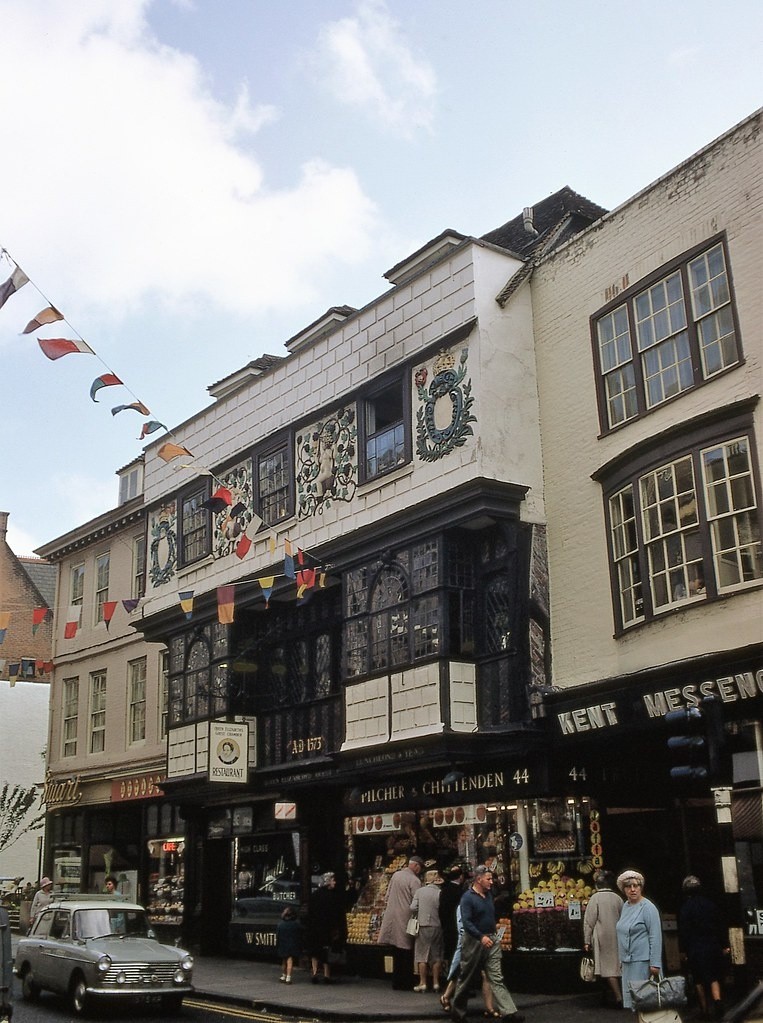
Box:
[513,907,585,948]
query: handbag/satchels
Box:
[405,914,420,936]
[580,951,597,982]
[636,1006,682,1023]
[628,970,688,1011]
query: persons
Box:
[615,869,661,1014]
[582,870,625,1010]
[275,907,297,984]
[438,865,477,999]
[677,876,730,1021]
[22,882,31,897]
[377,856,425,991]
[410,870,443,994]
[451,864,526,1023]
[301,871,347,985]
[30,877,53,934]
[238,864,251,890]
[104,876,125,934]
[7,879,19,891]
[440,878,502,1018]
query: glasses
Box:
[625,884,639,888]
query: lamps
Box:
[444,764,464,784]
[230,649,256,672]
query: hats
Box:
[425,870,444,884]
[411,856,426,868]
[39,877,53,888]
[616,870,644,891]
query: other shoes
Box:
[286,977,291,984]
[501,1012,525,1022]
[279,976,286,981]
[311,976,319,984]
[323,977,332,984]
[451,1015,469,1023]
[714,1000,724,1018]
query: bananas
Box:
[577,860,593,873]
[529,860,566,878]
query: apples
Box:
[495,918,512,951]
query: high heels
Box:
[433,984,439,993]
[414,984,426,993]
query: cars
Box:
[12,892,195,1018]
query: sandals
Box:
[484,1009,500,1019]
[440,996,451,1012]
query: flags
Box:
[0,265,305,580]
[0,564,328,644]
[0,657,54,687]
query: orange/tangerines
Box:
[346,912,371,939]
[513,874,595,910]
[385,856,400,873]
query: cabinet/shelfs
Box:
[147,880,185,924]
